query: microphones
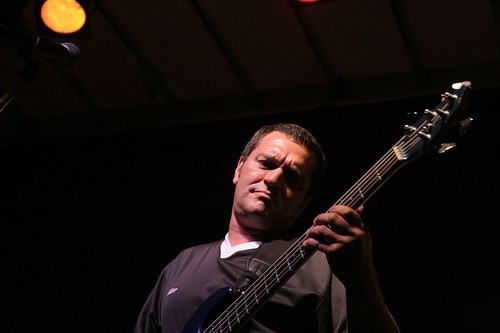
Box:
[0,24,81,68]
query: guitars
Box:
[180,81,474,333]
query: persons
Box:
[134,123,402,333]
